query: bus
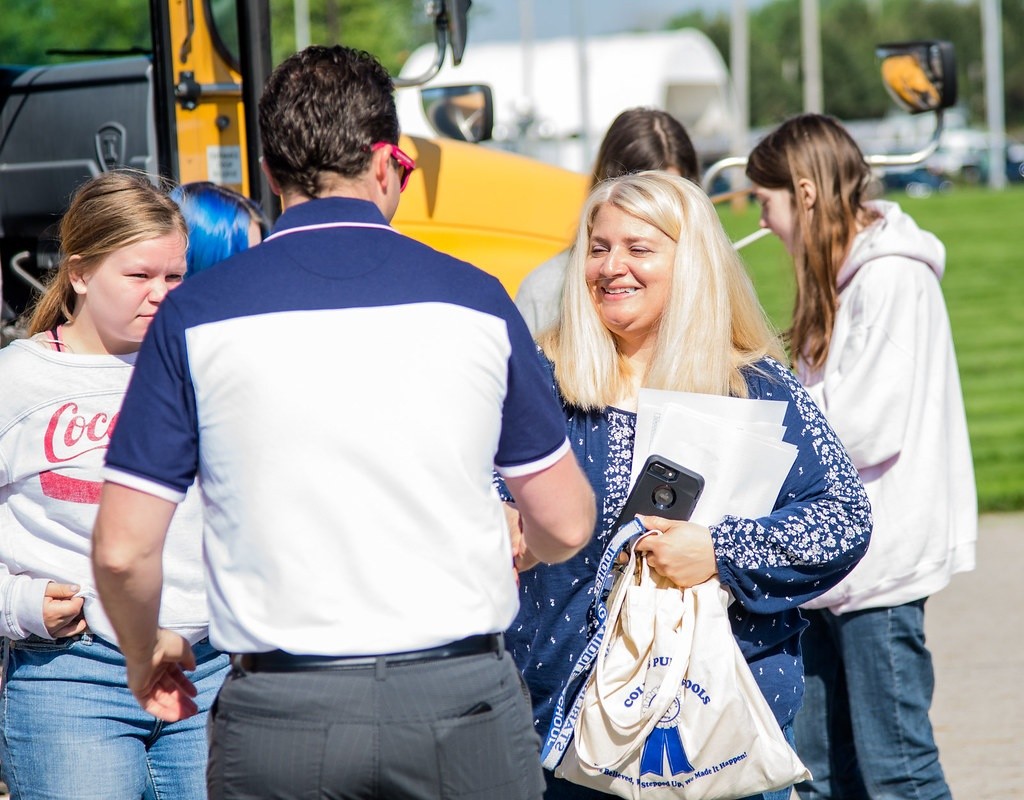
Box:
[0,0,960,344]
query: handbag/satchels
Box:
[541,511,813,800]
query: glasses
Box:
[362,141,418,192]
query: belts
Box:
[232,632,508,672]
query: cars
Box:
[879,134,1024,197]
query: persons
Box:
[515,107,700,340]
[0,172,189,800]
[492,170,873,800]
[89,43,597,800]
[744,112,979,800]
[166,182,264,282]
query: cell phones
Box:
[600,455,704,559]
